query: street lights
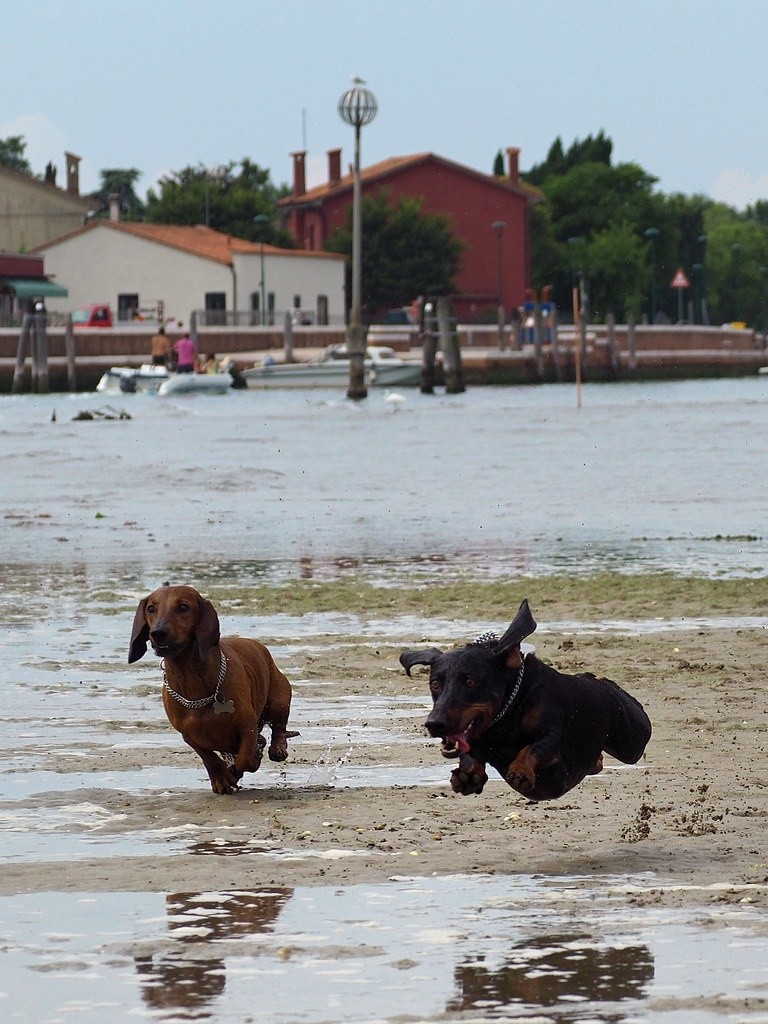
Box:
[338,76,379,401]
[491,218,509,352]
[645,225,661,326]
[254,212,269,326]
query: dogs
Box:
[399,597,652,805]
[127,586,302,795]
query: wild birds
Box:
[380,389,408,414]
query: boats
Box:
[93,363,235,398]
[238,344,423,391]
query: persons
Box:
[201,350,219,374]
[172,333,195,373]
[151,328,171,368]
